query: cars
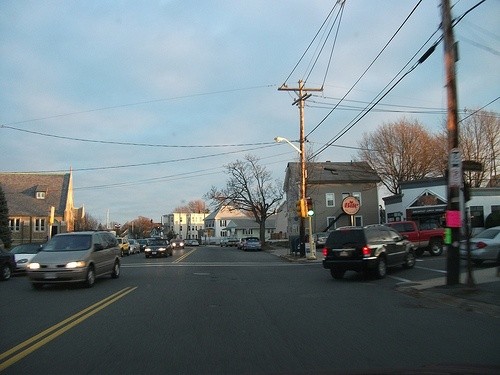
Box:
[0,243,45,281]
[311,232,328,246]
[219,236,262,251]
[460,226,500,266]
[116,237,200,259]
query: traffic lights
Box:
[294,196,314,218]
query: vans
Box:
[24,230,121,289]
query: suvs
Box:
[321,223,418,280]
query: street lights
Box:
[274,136,307,258]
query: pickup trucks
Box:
[381,220,447,257]
